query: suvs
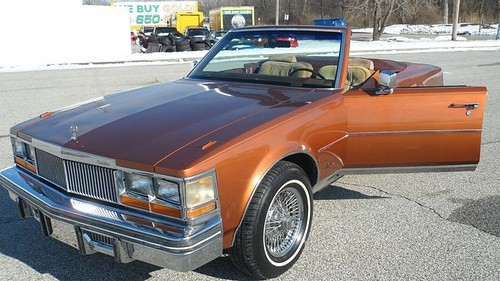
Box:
[138,24,155,48]
[183,26,210,40]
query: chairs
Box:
[259,61,314,79]
[267,56,297,63]
[347,58,374,73]
[317,65,370,89]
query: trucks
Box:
[313,18,347,28]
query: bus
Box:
[207,5,255,31]
[166,11,204,36]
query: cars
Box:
[151,26,178,37]
[255,33,298,48]
[206,30,241,50]
[1,25,489,280]
[131,32,137,40]
